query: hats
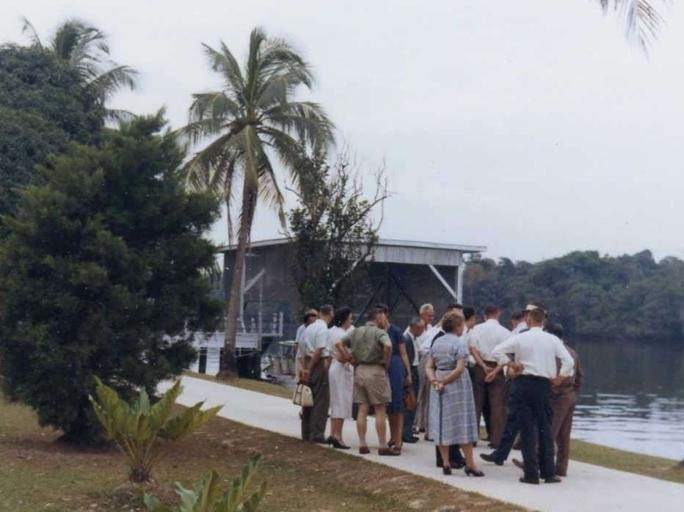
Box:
[522,303,548,318]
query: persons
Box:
[404,301,581,485]
[295,304,412,456]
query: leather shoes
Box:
[402,426,434,443]
[481,454,503,465]
[513,459,566,483]
[378,440,402,455]
[359,447,369,454]
[443,466,451,475]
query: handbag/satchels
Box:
[404,390,416,410]
[291,384,314,407]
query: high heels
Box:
[465,466,484,476]
[328,436,351,449]
